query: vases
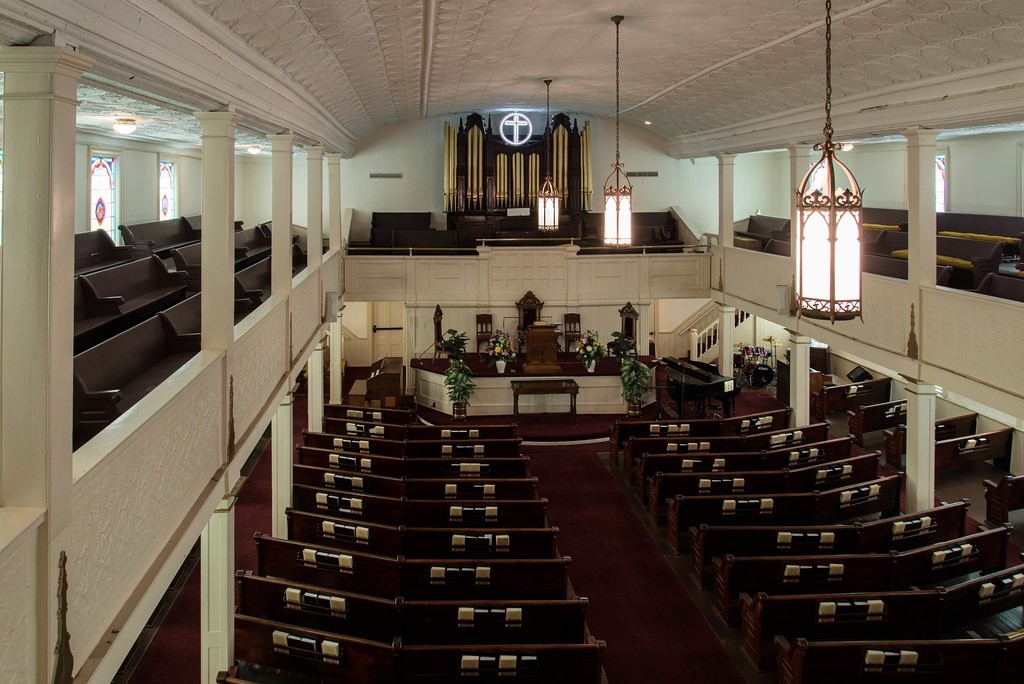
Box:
[495,359,506,373]
[585,361,595,373]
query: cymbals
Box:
[733,344,751,347]
[761,336,779,341]
[769,343,783,346]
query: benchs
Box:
[74,211,1024,684]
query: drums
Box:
[755,346,765,355]
[752,364,774,386]
[743,346,756,358]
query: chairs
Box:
[432,304,464,365]
[607,301,640,357]
[514,291,545,350]
[564,313,582,355]
[475,314,492,354]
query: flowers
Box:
[485,328,521,362]
[576,328,606,369]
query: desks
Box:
[511,379,580,415]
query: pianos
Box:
[364,356,404,408]
[663,355,743,418]
[777,358,833,416]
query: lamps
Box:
[537,78,560,232]
[795,0,866,325]
[602,16,634,247]
[113,118,136,134]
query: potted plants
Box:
[620,358,651,416]
[443,359,476,421]
